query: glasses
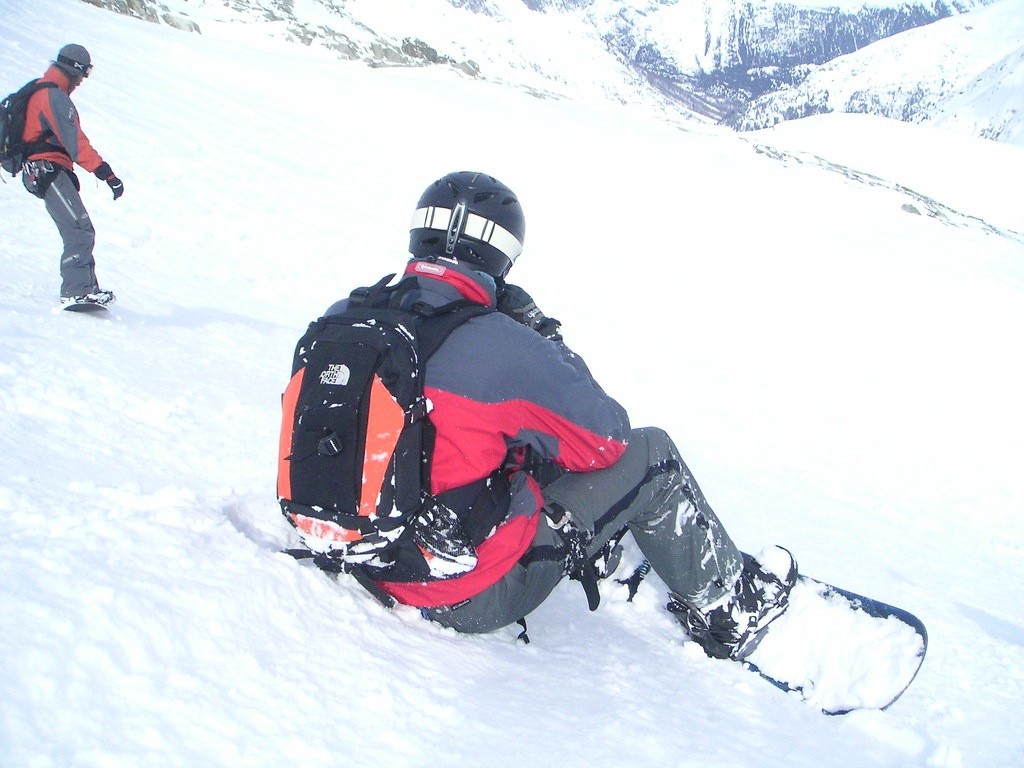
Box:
[57,54,93,78]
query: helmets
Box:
[408,170,525,280]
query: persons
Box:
[20,44,125,310]
[274,171,799,663]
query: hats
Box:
[55,44,91,77]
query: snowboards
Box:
[613,528,929,717]
[62,291,109,313]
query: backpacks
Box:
[0,78,69,173]
[276,273,511,576]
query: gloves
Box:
[106,173,124,201]
[496,283,562,338]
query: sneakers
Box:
[567,522,629,581]
[60,289,116,307]
[689,544,798,661]
